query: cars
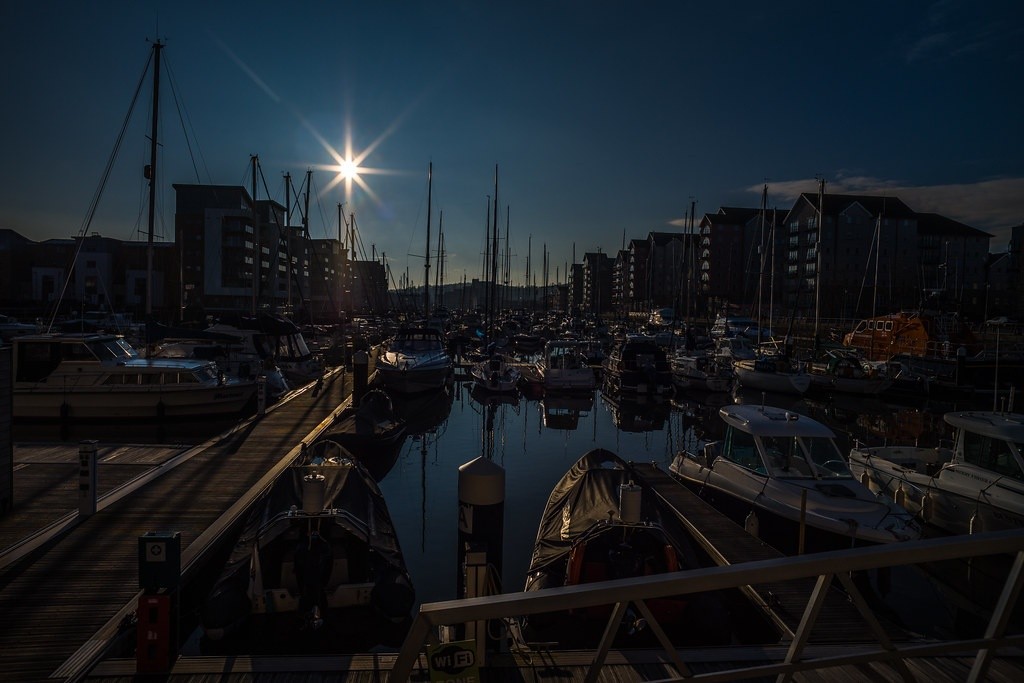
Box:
[985,315,1009,327]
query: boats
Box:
[669,389,922,554]
[321,387,409,449]
[196,436,416,647]
[849,409,1024,534]
[519,443,782,646]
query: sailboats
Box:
[0,6,1023,422]
